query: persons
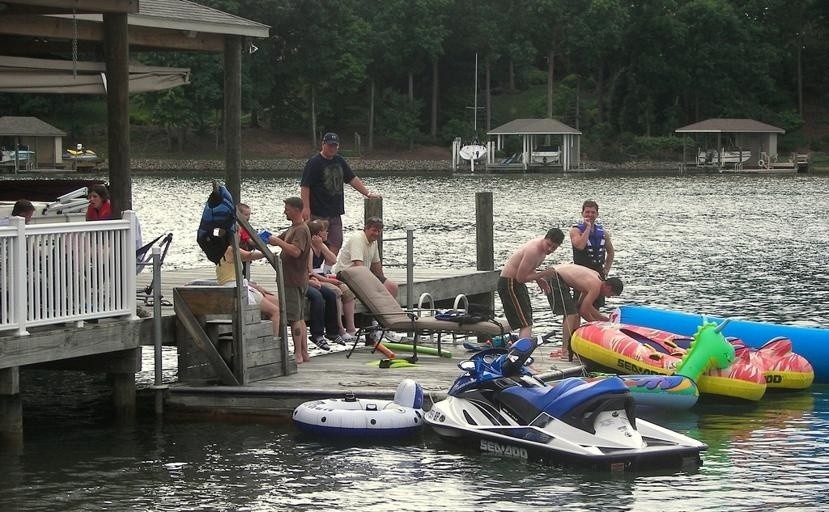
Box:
[498,200,623,362]
[0,198,36,227]
[86,183,111,220]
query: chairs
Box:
[336,264,514,364]
[83,231,173,322]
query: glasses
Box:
[370,218,383,223]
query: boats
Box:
[533,144,562,164]
[289,377,425,442]
[567,303,829,412]
[698,149,751,163]
[792,152,811,170]
[8,151,34,162]
[61,143,104,168]
[459,144,487,164]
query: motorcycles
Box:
[425,330,707,476]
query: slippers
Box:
[325,333,346,345]
[309,336,330,351]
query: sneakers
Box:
[370,322,400,343]
[343,331,363,342]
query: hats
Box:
[322,132,340,146]
